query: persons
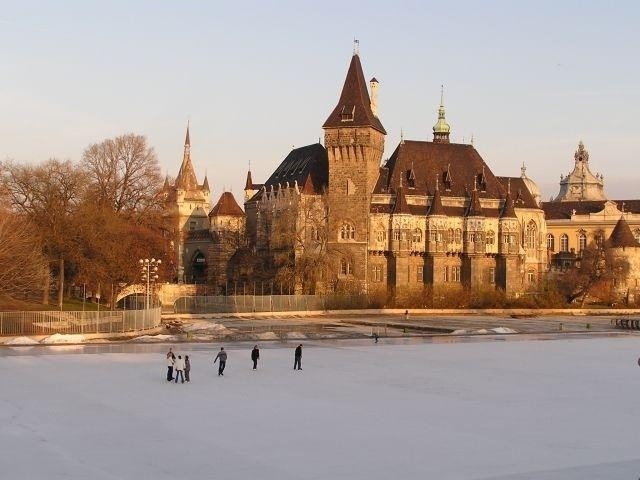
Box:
[174,355,186,384]
[251,344,260,371]
[374,333,380,343]
[213,347,228,376]
[166,352,177,381]
[293,343,303,371]
[166,347,176,359]
[184,355,191,383]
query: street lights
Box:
[137,257,162,309]
